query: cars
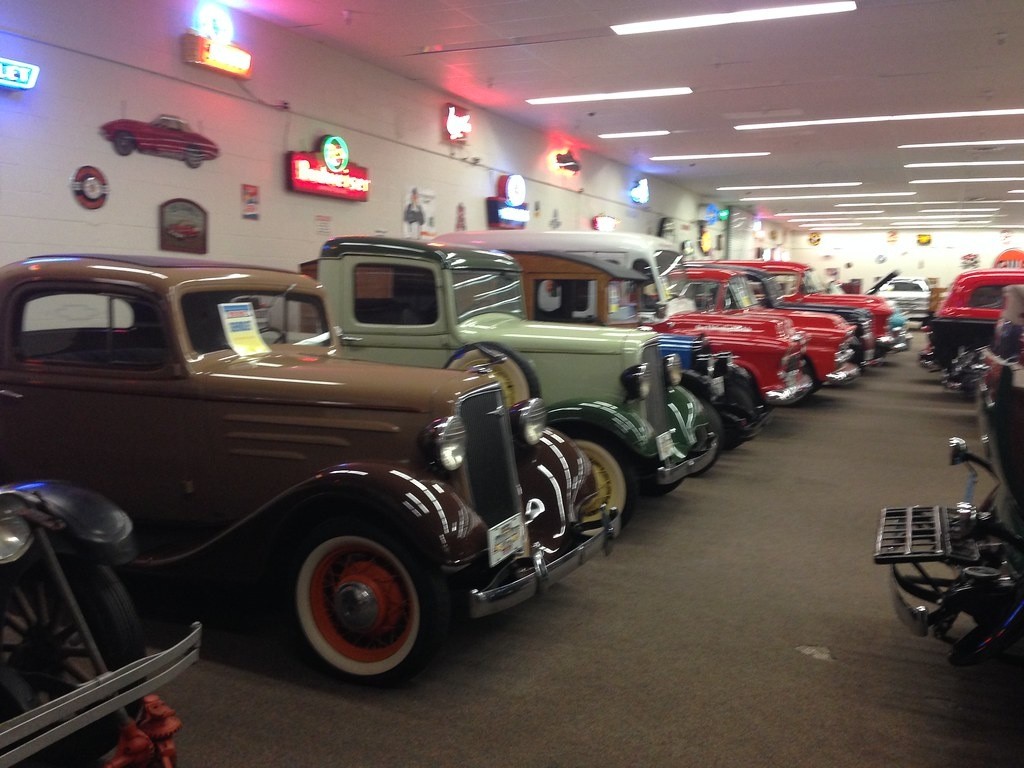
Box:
[0,476,205,768]
[0,250,621,694]
[237,233,720,545]
[378,228,1024,677]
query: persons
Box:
[993,284,1024,365]
